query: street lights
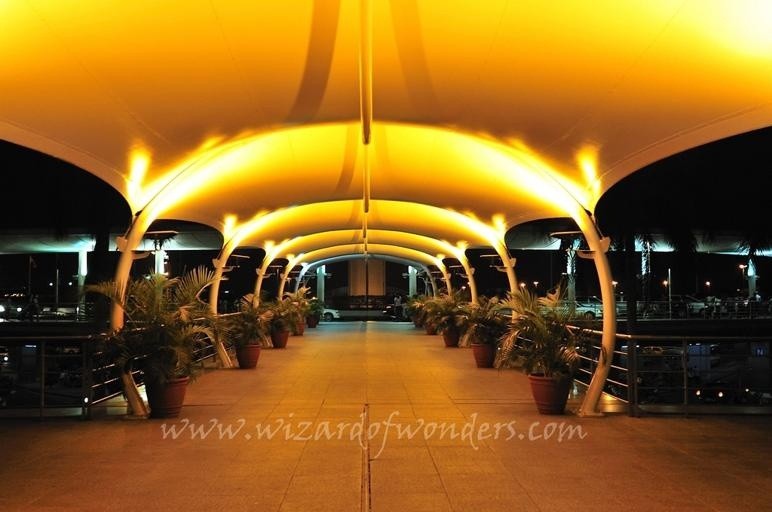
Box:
[738,264,748,293]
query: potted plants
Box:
[407,294,471,347]
[494,279,601,416]
[452,295,514,368]
[264,288,322,348]
[75,265,229,418]
[222,293,272,369]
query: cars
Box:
[638,344,684,358]
[321,307,341,322]
[0,346,11,365]
[485,295,719,319]
[382,303,408,322]
[55,343,81,354]
